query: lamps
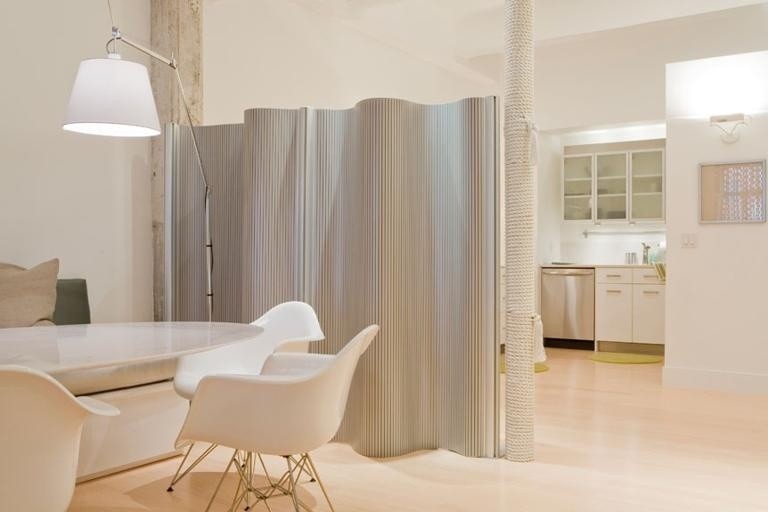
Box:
[63,25,215,323]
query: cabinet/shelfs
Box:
[564,148,663,224]
[596,267,666,346]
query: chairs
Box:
[168,325,381,512]
[174,301,325,494]
[0,365,123,512]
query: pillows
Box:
[1,253,63,330]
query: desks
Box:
[1,320,263,486]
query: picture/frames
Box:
[697,159,766,226]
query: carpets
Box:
[588,350,663,364]
[500,352,547,374]
